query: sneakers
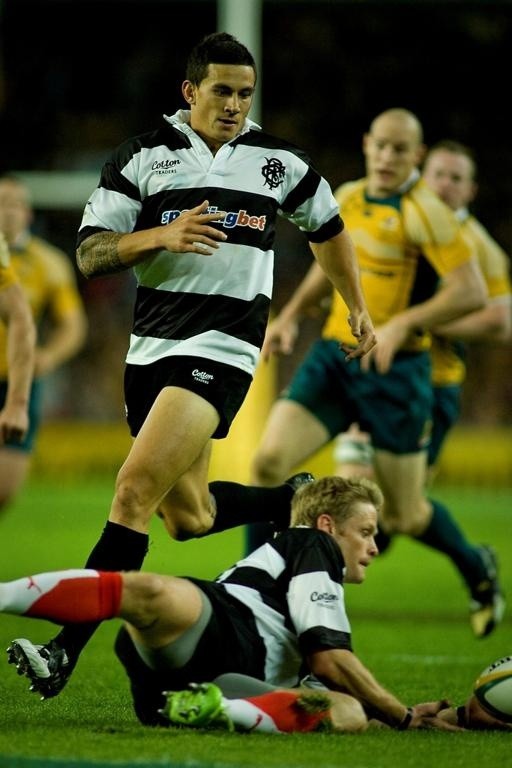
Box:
[470,544,504,638]
[160,680,224,727]
[6,637,69,700]
[286,472,313,490]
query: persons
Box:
[255,109,506,638]
[0,251,37,441]
[437,658,512,731]
[5,30,376,700]
[1,178,89,511]
[0,472,469,732]
[332,138,512,557]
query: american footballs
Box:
[473,656,512,724]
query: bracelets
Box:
[399,708,413,731]
